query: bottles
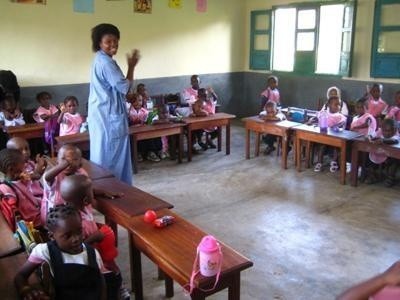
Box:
[319,111,329,133]
[197,236,222,277]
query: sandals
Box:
[330,161,339,172]
[314,163,323,172]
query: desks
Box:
[240,106,400,188]
[93,178,174,248]
[55,132,90,160]
[0,253,40,300]
[50,158,116,180]
[7,123,45,139]
[119,208,253,299]
[181,112,236,161]
[0,210,23,256]
[129,121,187,174]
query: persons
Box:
[126,75,218,162]
[0,70,89,155]
[314,82,400,187]
[259,75,292,156]
[88,23,140,185]
[0,137,130,299]
[334,261,400,300]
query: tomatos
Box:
[143,211,157,224]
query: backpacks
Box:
[0,195,21,233]
[44,117,59,143]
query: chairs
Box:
[41,261,58,300]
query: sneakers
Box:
[345,162,351,174]
[208,140,216,148]
[199,142,207,150]
[166,152,170,158]
[192,144,202,153]
[136,153,143,161]
[264,146,276,155]
[287,146,292,154]
[148,151,160,162]
[160,152,166,160]
